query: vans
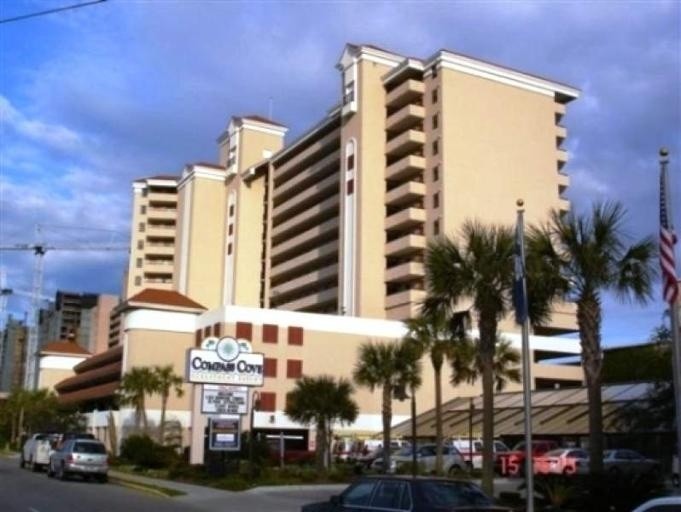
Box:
[17,429,110,482]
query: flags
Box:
[511,214,528,325]
[658,162,679,304]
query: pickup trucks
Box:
[493,441,569,478]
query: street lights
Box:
[247,387,262,457]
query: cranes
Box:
[0,218,130,394]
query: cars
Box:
[326,437,512,475]
[300,475,520,512]
[576,449,663,478]
[631,495,680,511]
[531,448,586,475]
